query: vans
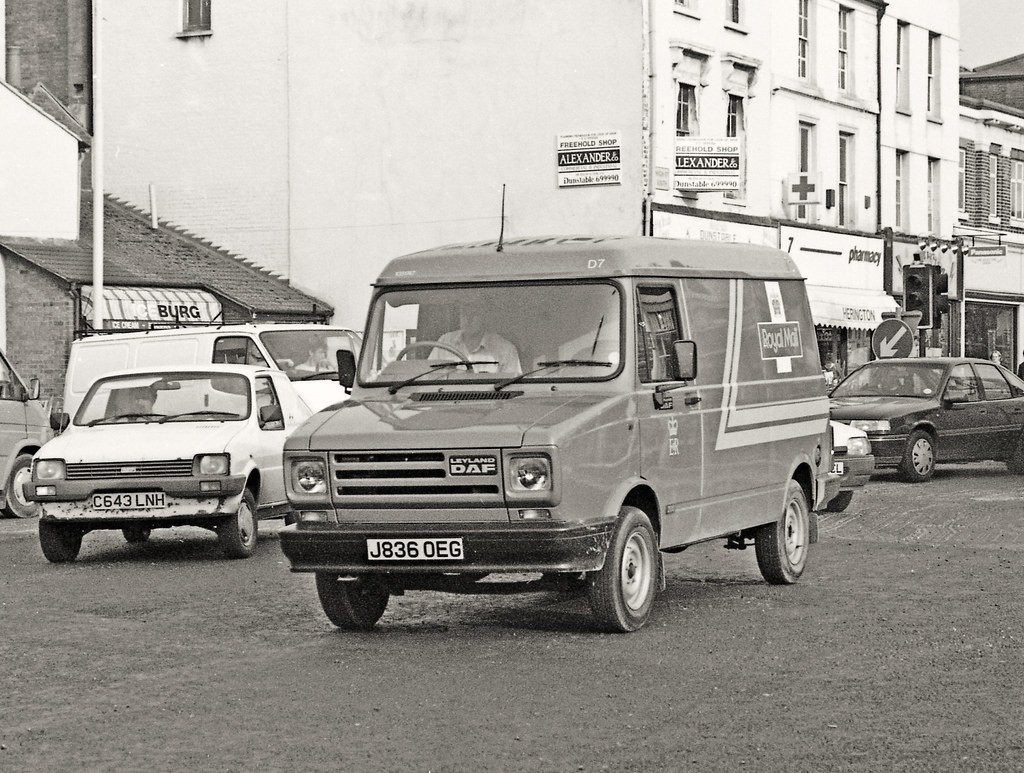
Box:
[0,348,55,518]
[275,184,841,632]
[63,303,389,422]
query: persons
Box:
[429,291,523,372]
[885,370,906,394]
[134,388,158,414]
[236,343,295,367]
[991,351,1008,369]
[1018,350,1024,381]
[296,341,333,372]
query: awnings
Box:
[81,284,222,335]
[806,287,901,331]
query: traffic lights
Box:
[903,265,949,328]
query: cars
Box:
[828,357,1024,482]
[822,420,875,512]
[22,364,312,564]
